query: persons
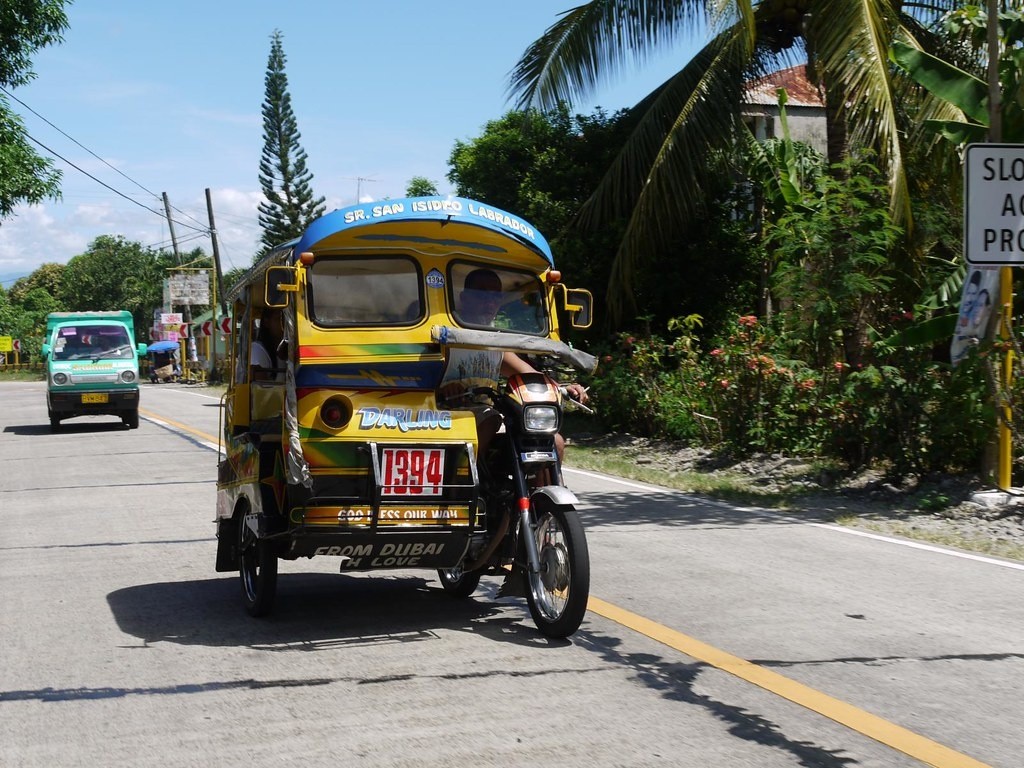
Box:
[418,268,589,487]
[166,358,182,383]
[250,309,286,517]
[149,361,159,384]
[91,335,112,354]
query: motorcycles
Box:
[211,197,599,642]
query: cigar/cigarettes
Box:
[575,386,590,399]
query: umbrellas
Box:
[147,341,181,381]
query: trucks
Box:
[42,310,147,429]
